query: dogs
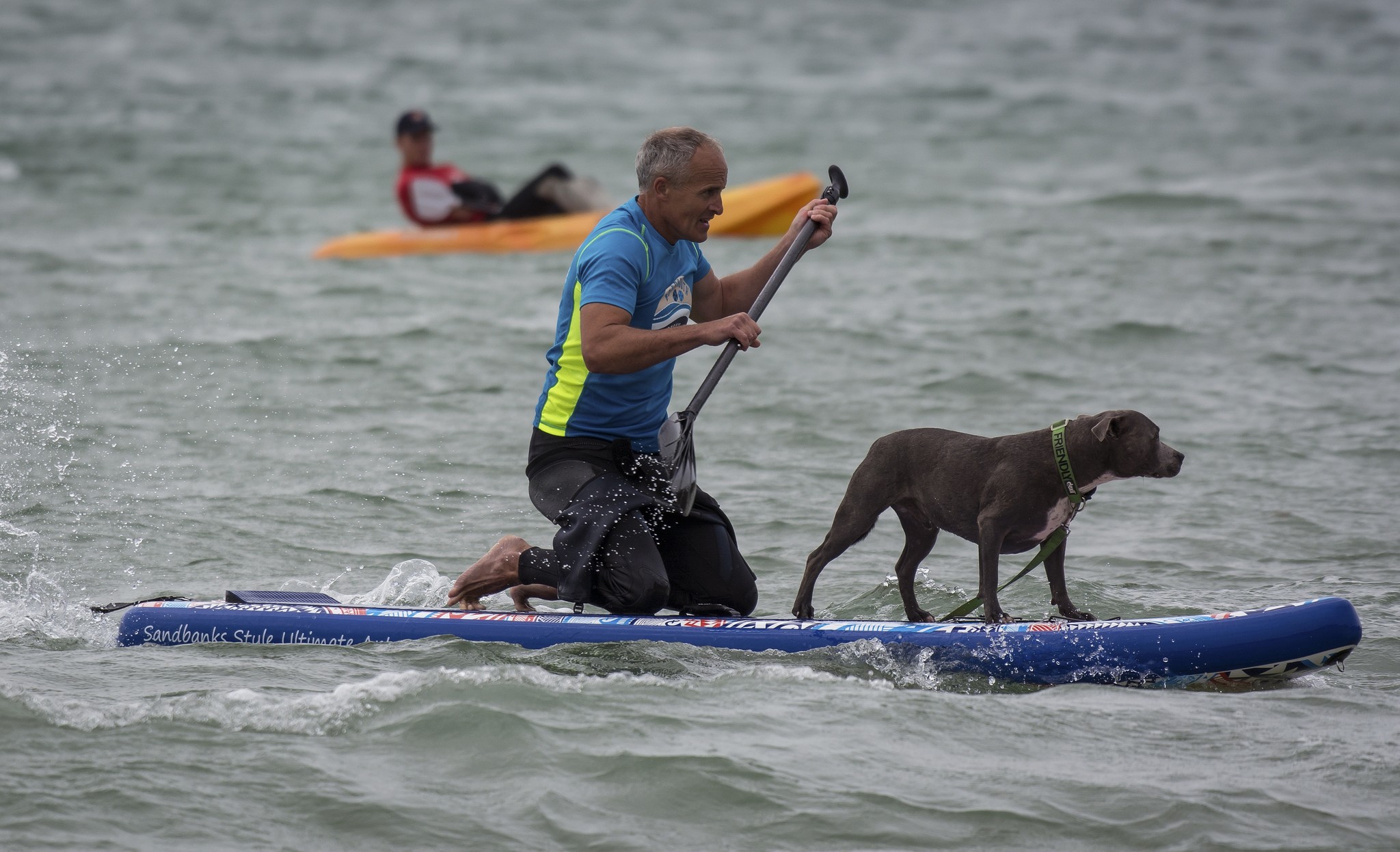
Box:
[789,405,1187,626]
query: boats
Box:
[310,173,825,261]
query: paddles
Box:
[655,163,850,518]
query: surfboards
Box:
[112,587,1365,693]
[309,172,822,259]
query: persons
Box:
[396,110,611,227]
[445,129,840,624]
